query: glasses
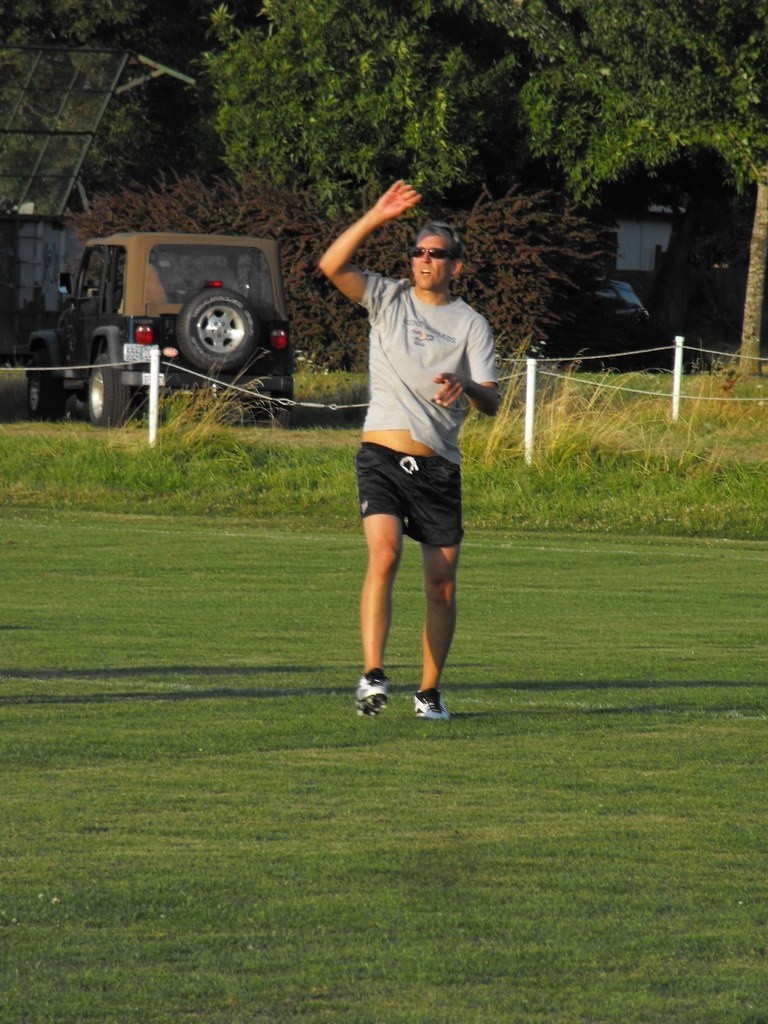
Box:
[408,247,456,260]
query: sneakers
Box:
[414,688,449,718]
[355,668,388,716]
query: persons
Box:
[320,180,499,722]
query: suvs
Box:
[22,231,295,431]
